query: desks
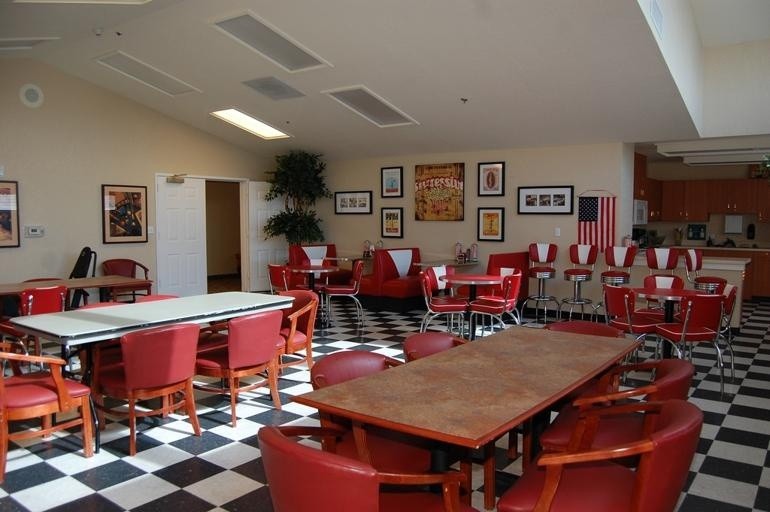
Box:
[10,290,295,454]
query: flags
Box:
[577,197,615,254]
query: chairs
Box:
[194,309,289,427]
[91,324,201,456]
[1,246,179,373]
[0,343,93,486]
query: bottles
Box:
[363,251,370,260]
[456,254,466,264]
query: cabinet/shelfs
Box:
[710,180,747,213]
[748,179,770,213]
[662,182,708,223]
[634,152,661,222]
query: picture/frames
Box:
[0,180,20,248]
[101,184,148,244]
[517,185,574,215]
[415,163,464,221]
[478,161,505,196]
[381,166,402,198]
[334,191,372,214]
[477,207,505,242]
[381,207,403,238]
